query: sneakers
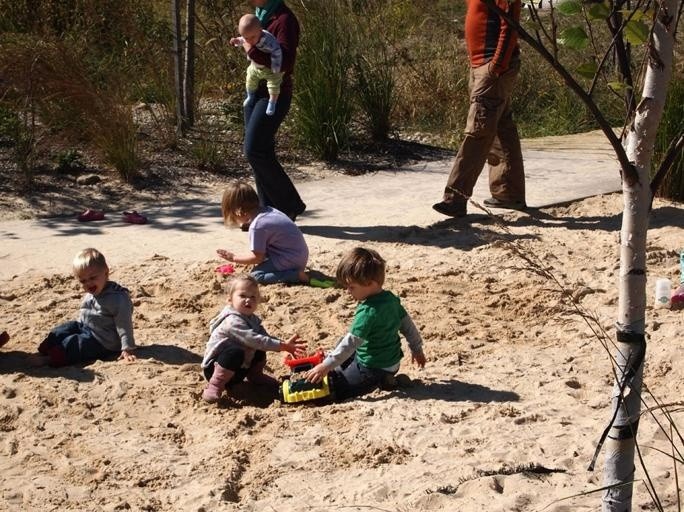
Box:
[383,373,411,390]
[484,198,525,210]
[433,202,467,216]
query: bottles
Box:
[656,278,671,310]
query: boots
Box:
[203,362,234,402]
[248,360,273,384]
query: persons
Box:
[216,181,310,285]
[200,275,308,404]
[432,0,526,219]
[28,248,138,368]
[304,247,427,406]
[238,0,307,230]
[230,14,282,116]
